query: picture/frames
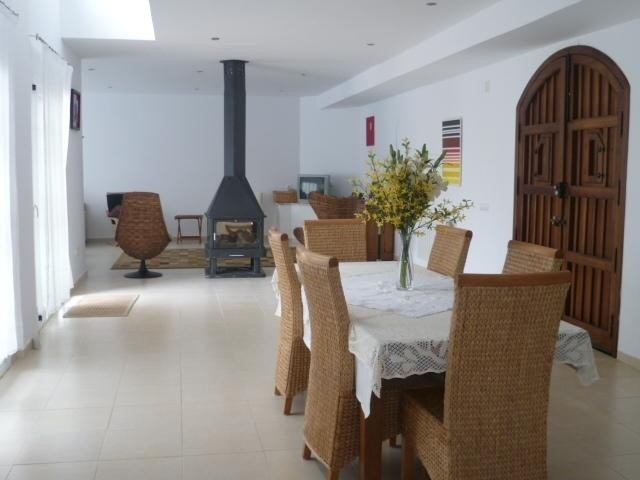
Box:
[70,89,81,129]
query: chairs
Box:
[426,222,475,279]
[290,253,406,477]
[270,227,314,415]
[503,239,562,279]
[301,220,369,262]
[116,192,175,279]
[398,271,573,476]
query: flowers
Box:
[349,139,474,289]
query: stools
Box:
[175,215,204,246]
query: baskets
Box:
[273,188,297,204]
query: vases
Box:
[397,227,413,291]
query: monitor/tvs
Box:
[296,174,330,203]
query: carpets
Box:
[64,293,141,319]
[111,249,297,267]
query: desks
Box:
[272,260,588,480]
